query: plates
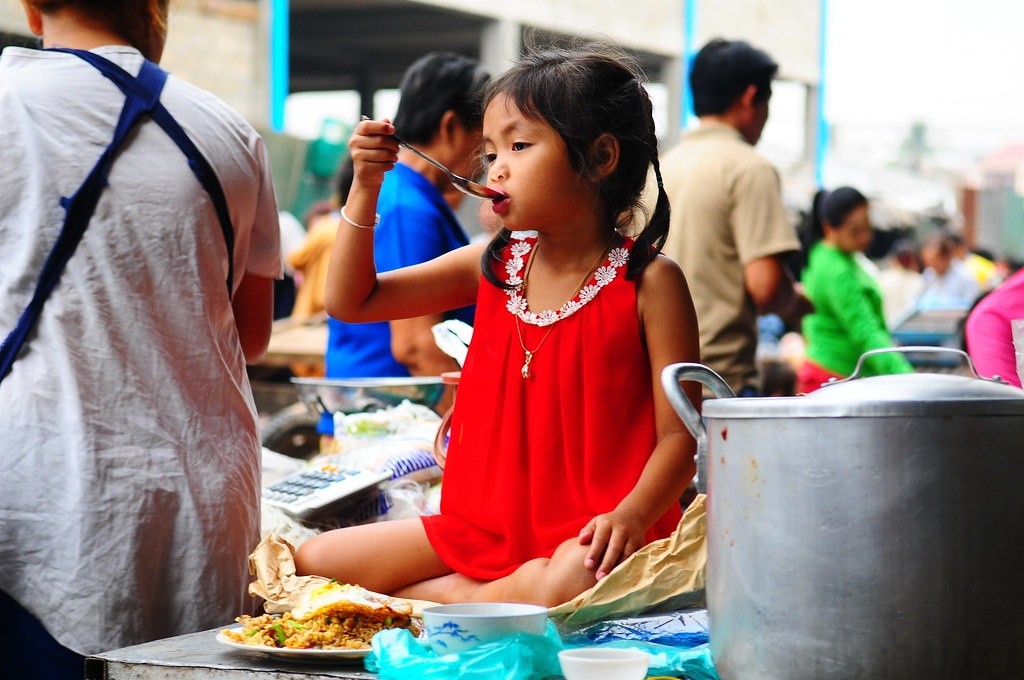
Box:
[216,627,371,659]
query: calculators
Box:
[264,458,396,516]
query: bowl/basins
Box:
[423,602,549,657]
[289,377,445,416]
[558,648,649,680]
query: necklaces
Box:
[516,231,618,378]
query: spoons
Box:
[362,115,506,200]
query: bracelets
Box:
[340,206,380,229]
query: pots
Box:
[659,345,1023,680]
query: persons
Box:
[796,188,913,394]
[0,0,284,680]
[274,51,502,458]
[882,232,1024,388]
[294,50,703,607]
[627,39,801,398]
